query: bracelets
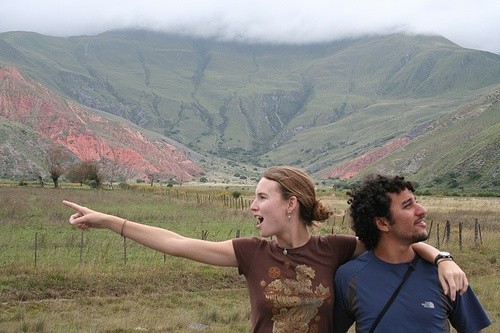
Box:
[120,219,127,236]
[437,258,455,266]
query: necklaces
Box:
[277,244,288,255]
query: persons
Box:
[333,173,492,333]
[61,166,469,333]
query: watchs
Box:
[435,251,454,268]
